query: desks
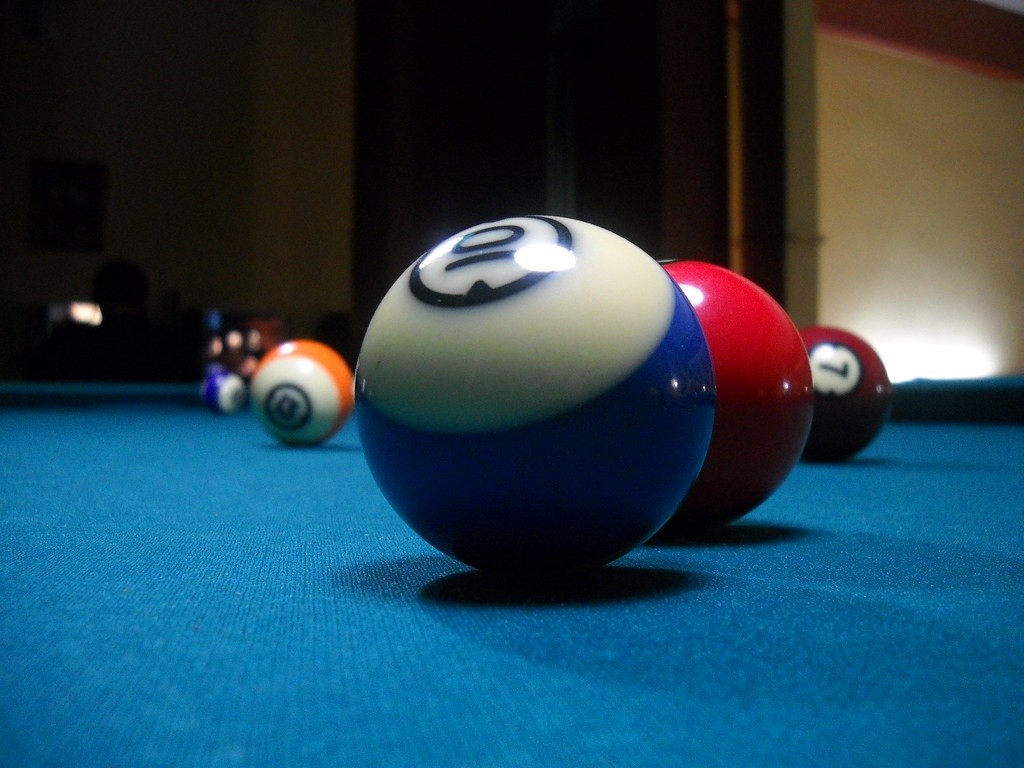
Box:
[0,371,1024,768]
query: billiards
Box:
[202,212,889,590]
